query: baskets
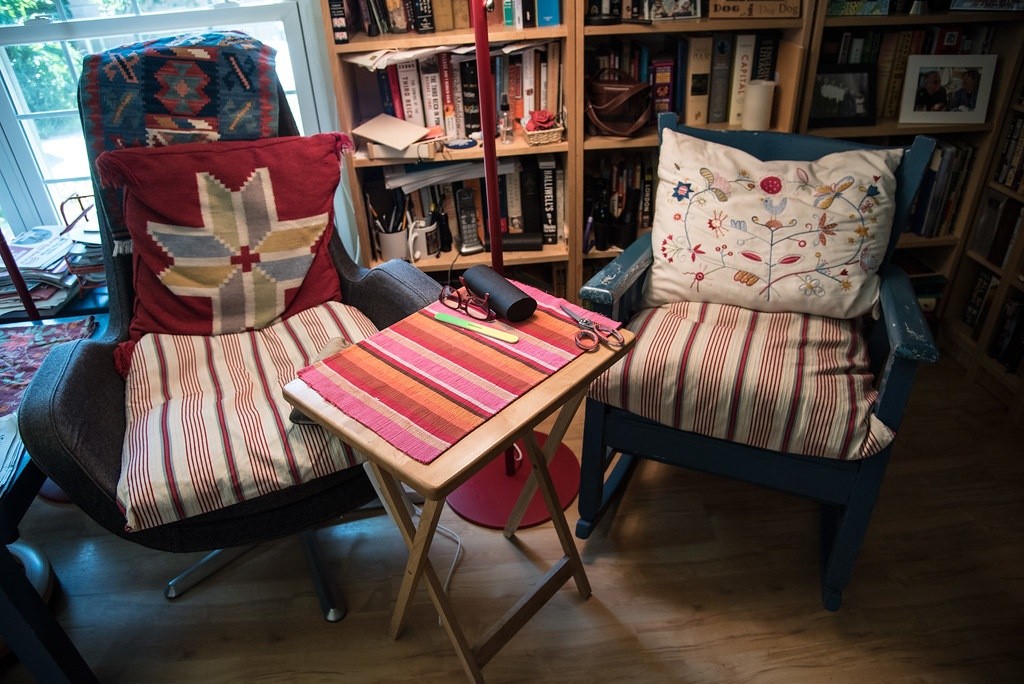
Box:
[521,119,564,146]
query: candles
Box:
[741,79,774,132]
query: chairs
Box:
[575,114,937,623]
[17,28,452,624]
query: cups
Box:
[374,221,440,264]
[743,80,775,131]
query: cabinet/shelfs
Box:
[794,0,1024,320]
[320,0,816,310]
[913,59,1024,418]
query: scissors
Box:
[560,306,625,353]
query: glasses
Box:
[439,277,496,321]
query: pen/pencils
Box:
[369,198,445,233]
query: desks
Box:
[276,279,637,684]
[0,314,109,684]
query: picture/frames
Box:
[643,0,703,20]
[898,54,997,125]
[808,62,879,127]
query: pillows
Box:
[637,127,905,322]
[93,131,354,378]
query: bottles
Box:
[592,196,610,251]
[498,94,514,144]
[440,215,453,252]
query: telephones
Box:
[456,187,484,255]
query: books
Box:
[827,0,1024,17]
[586,0,803,26]
[900,134,974,238]
[384,159,567,246]
[820,27,997,119]
[371,42,563,143]
[991,74,1024,194]
[329,0,563,45]
[590,31,778,127]
[958,265,1024,379]
[965,184,1024,271]
[585,158,655,250]
[0,256,80,305]
[900,258,942,312]
[69,216,106,284]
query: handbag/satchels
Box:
[584,67,653,138]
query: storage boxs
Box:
[708,0,800,18]
[366,140,436,162]
